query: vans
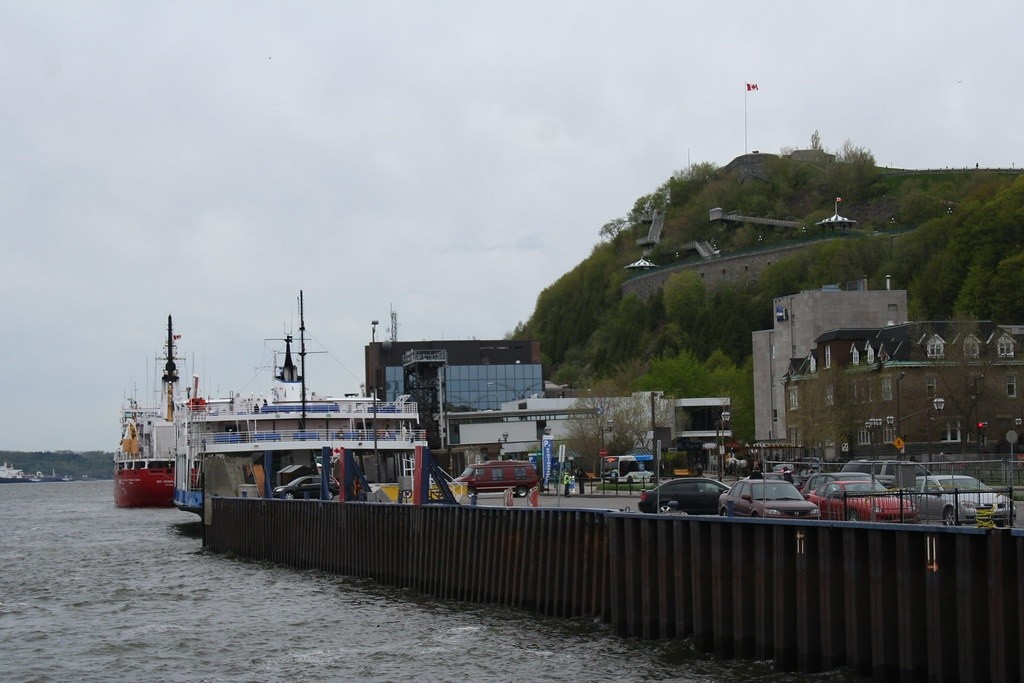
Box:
[450,458,538,498]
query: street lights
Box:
[651,391,658,483]
[975,374,984,447]
[896,371,906,460]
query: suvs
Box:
[273,473,340,501]
[839,458,934,490]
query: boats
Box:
[0,460,75,483]
[113,314,199,509]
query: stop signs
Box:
[599,448,608,458]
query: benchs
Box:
[331,431,396,441]
[292,432,319,441]
[213,434,241,443]
[254,433,281,442]
[260,404,339,413]
[368,406,402,414]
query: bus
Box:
[599,455,655,483]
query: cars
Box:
[717,479,822,522]
[638,476,733,516]
[801,480,920,524]
[892,474,1018,527]
[743,456,822,491]
[798,472,883,497]
